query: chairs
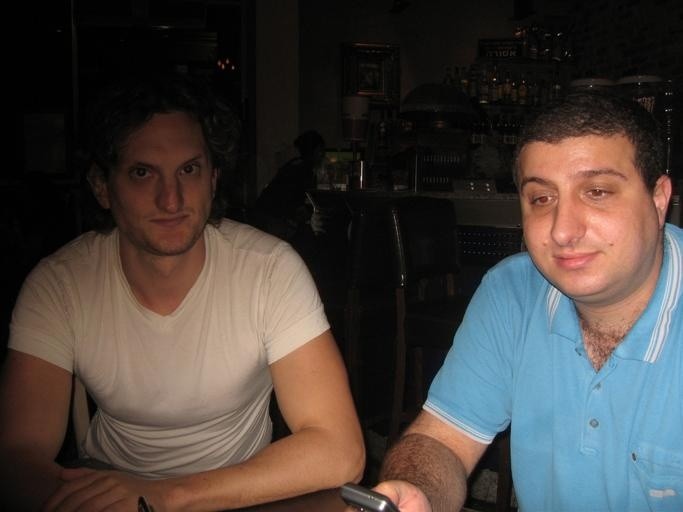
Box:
[386,196,473,450]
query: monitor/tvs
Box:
[312,148,366,191]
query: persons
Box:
[334,90,681,512]
[0,89,369,511]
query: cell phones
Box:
[339,482,401,511]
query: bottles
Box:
[443,68,563,160]
